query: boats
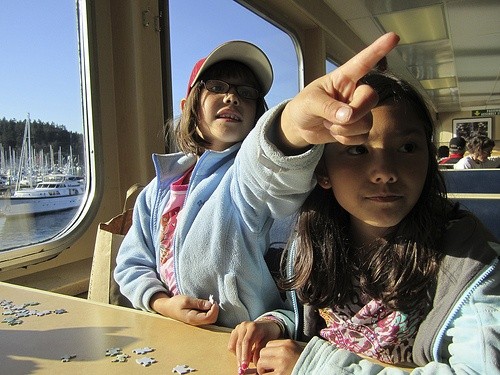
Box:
[9,174,85,199]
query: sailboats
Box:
[0,112,84,190]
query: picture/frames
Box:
[452,117,493,143]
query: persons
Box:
[113,31,401,329]
[227,72,500,375]
[453,134,500,169]
[430,137,468,169]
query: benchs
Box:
[438,164,500,243]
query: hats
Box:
[451,137,466,150]
[187,40,274,99]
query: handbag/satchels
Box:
[87,183,151,308]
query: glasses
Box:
[197,79,261,102]
[482,150,492,157]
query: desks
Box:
[0,282,415,375]
